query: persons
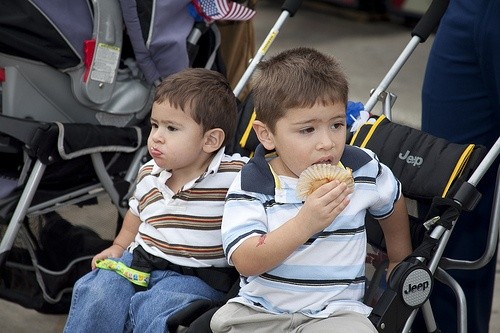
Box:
[210,46,419,332]
[60,66,252,333]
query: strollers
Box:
[1,0,499,333]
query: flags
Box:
[191,0,256,21]
